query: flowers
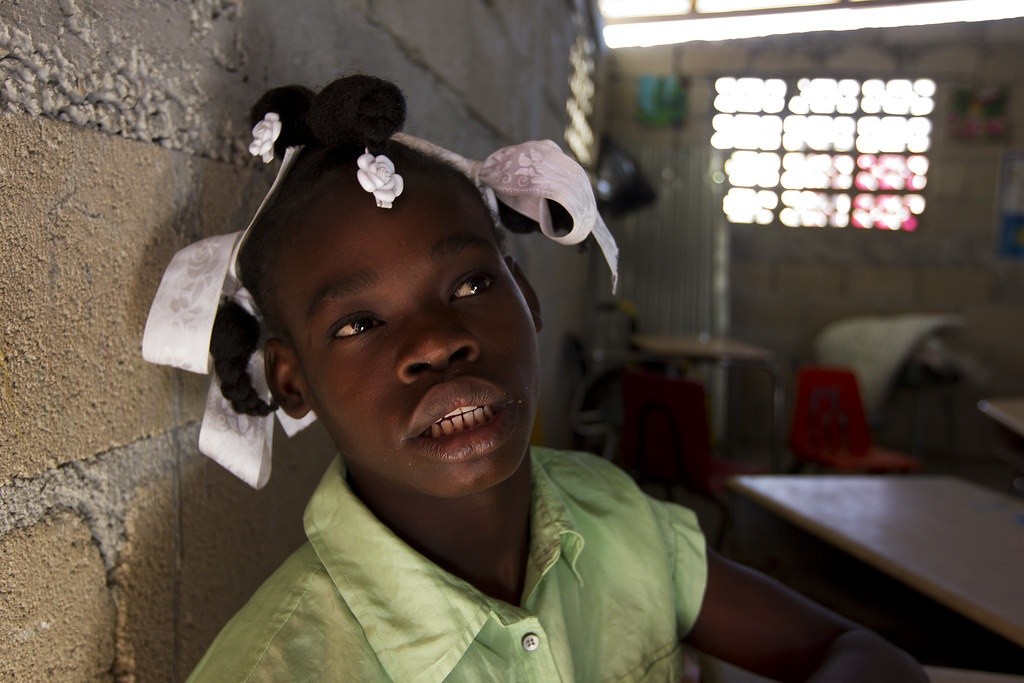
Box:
[357,154,404,209]
[248,113,281,164]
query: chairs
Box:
[787,370,922,475]
[618,370,769,552]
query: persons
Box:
[179,75,935,683]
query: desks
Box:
[734,475,1024,646]
[981,401,1024,436]
[636,336,773,439]
